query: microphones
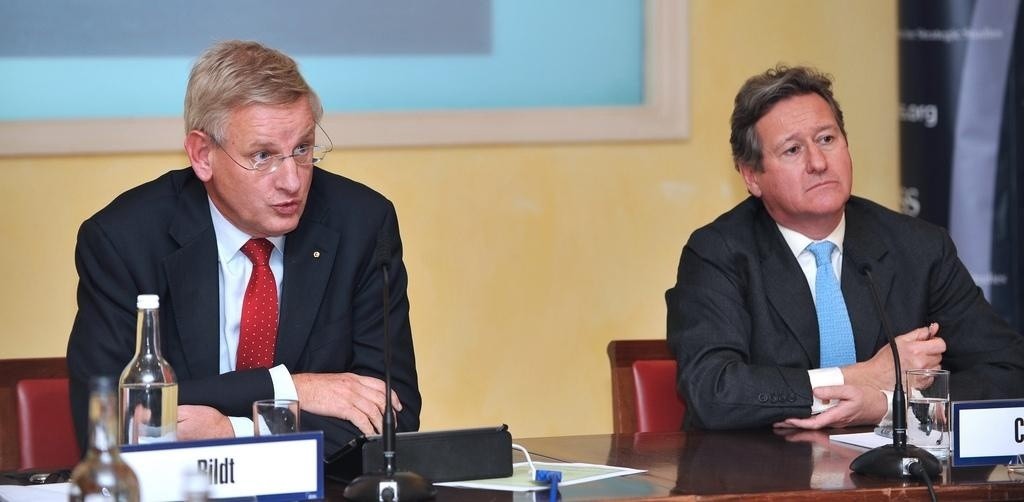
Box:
[343,245,438,502]
[847,247,944,479]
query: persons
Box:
[66,39,423,475]
[666,63,1024,441]
[667,424,864,498]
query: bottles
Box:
[71,379,140,502]
[119,293,178,447]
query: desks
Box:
[71,428,1022,502]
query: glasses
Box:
[208,122,335,177]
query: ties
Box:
[806,242,858,370]
[237,238,278,371]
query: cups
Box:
[253,398,301,435]
[904,368,951,461]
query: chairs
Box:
[0,358,81,484]
[604,340,684,431]
